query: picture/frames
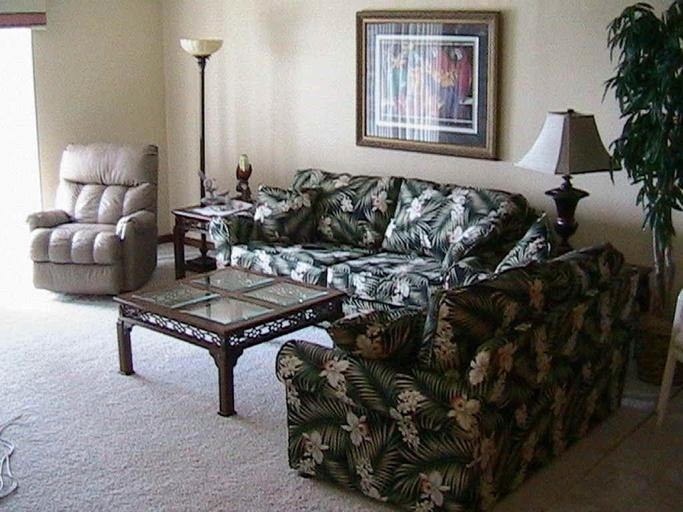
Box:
[354,11,501,164]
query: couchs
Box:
[207,167,561,328]
[276,241,639,485]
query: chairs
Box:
[25,140,159,296]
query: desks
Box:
[620,262,653,360]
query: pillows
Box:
[382,177,511,262]
[494,213,559,274]
[441,257,494,290]
[325,306,419,363]
[250,182,324,247]
[290,169,402,254]
[439,194,529,269]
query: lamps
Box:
[514,108,624,251]
[179,36,225,272]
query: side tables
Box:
[171,195,256,280]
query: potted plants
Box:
[596,0,682,388]
